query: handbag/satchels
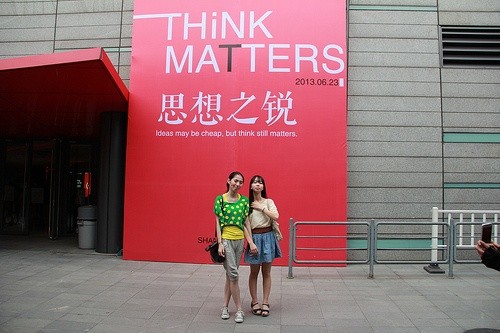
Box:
[205,241,226,264]
[267,198,283,241]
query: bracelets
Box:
[262,207,265,212]
[218,242,222,244]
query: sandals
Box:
[261,303,270,317]
[251,300,262,316]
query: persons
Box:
[476,239,500,271]
[244,175,283,317]
[213,171,259,323]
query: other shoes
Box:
[221,306,230,319]
[234,309,245,322]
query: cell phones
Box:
[482,223,492,244]
